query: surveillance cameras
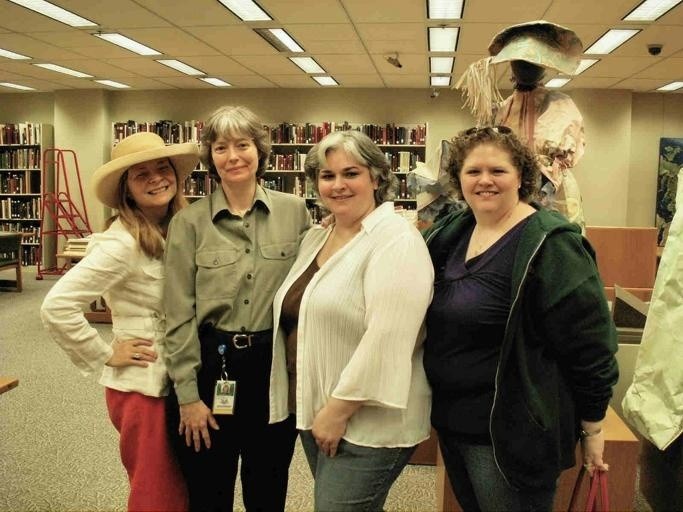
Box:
[388,56,403,68]
[430,88,441,98]
[650,44,663,55]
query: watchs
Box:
[578,428,603,440]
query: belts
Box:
[214,331,272,349]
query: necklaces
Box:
[471,214,523,256]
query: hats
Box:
[91,132,200,209]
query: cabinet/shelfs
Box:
[0,123,56,268]
[112,119,428,230]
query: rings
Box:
[583,462,593,467]
[133,352,140,358]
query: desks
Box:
[0,377,20,395]
[0,232,23,291]
[54,253,113,324]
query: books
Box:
[0,122,41,267]
[262,121,334,221]
[113,120,218,201]
[335,121,426,225]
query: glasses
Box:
[464,126,512,136]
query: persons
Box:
[160,104,313,511]
[266,129,438,512]
[221,385,229,393]
[421,124,614,512]
[34,132,199,511]
[450,18,589,246]
